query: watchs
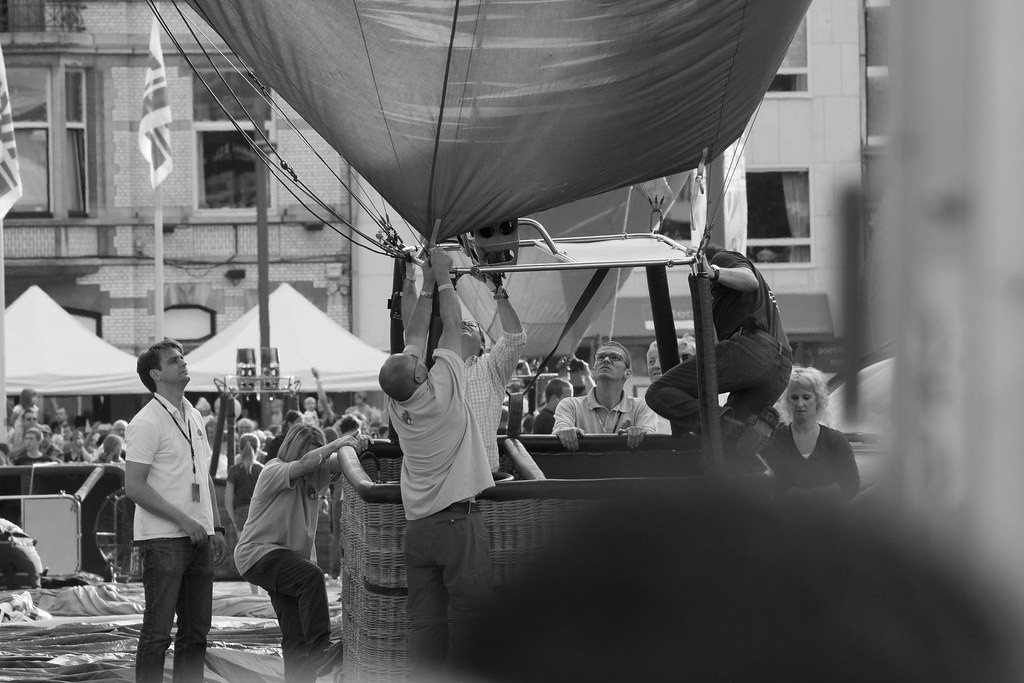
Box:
[709,263,721,282]
[493,289,509,301]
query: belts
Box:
[742,328,793,361]
[441,501,478,511]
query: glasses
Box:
[594,353,629,367]
[466,320,481,335]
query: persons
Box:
[402,248,528,482]
[379,247,501,682]
[495,331,711,454]
[762,365,860,507]
[125,339,230,683]
[644,244,797,487]
[3,362,384,478]
[232,421,375,682]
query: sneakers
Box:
[313,640,343,676]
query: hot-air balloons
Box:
[145,0,820,679]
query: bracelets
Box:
[214,526,226,536]
[420,290,433,298]
[436,283,455,291]
